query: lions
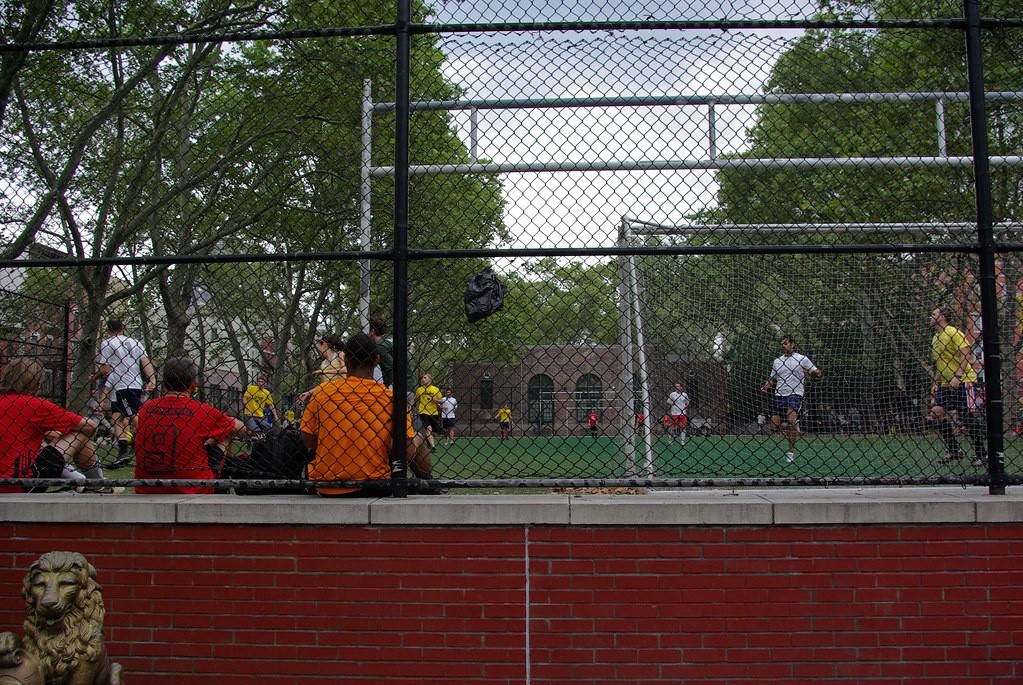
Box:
[0,551,128,685]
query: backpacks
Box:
[251,424,316,476]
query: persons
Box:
[635,411,669,437]
[667,383,690,446]
[760,335,822,462]
[758,412,765,436]
[588,410,598,438]
[0,316,458,498]
[495,404,513,439]
[928,305,991,467]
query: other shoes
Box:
[787,452,794,462]
[680,440,685,445]
[938,448,964,463]
[76,485,125,493]
[108,453,131,466]
[668,434,672,445]
[444,436,454,446]
[411,475,450,495]
[426,438,437,452]
[971,453,989,467]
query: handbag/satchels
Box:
[464,273,508,322]
[222,457,297,495]
